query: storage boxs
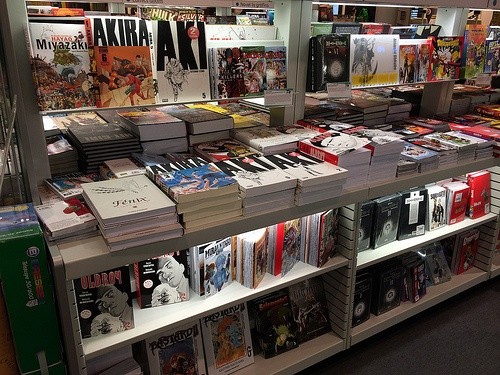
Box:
[0,201,67,375]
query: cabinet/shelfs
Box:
[1,0,371,374]
[294,1,499,349]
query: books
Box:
[304,3,500,95]
[359,169,490,252]
[25,1,288,116]
[92,274,333,375]
[352,229,480,328]
[71,205,339,339]
[32,101,500,253]
[42,83,425,178]
